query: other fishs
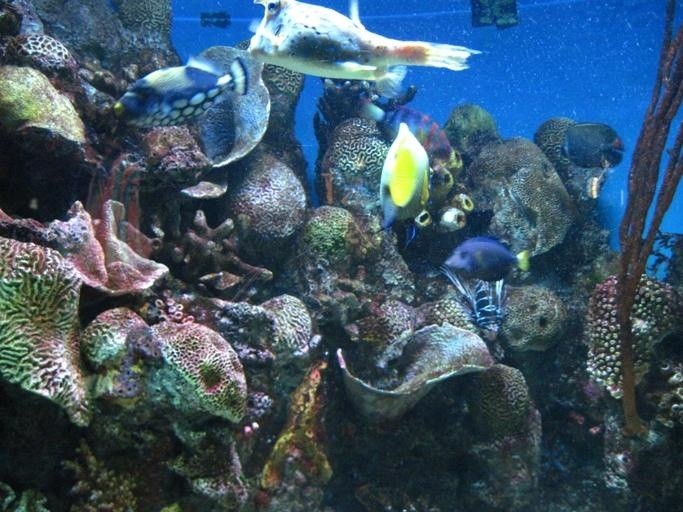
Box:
[357,101,463,170]
[190,46,270,168]
[245,0,483,100]
[559,122,625,169]
[112,53,250,130]
[379,122,431,252]
[444,236,531,283]
[441,264,506,340]
